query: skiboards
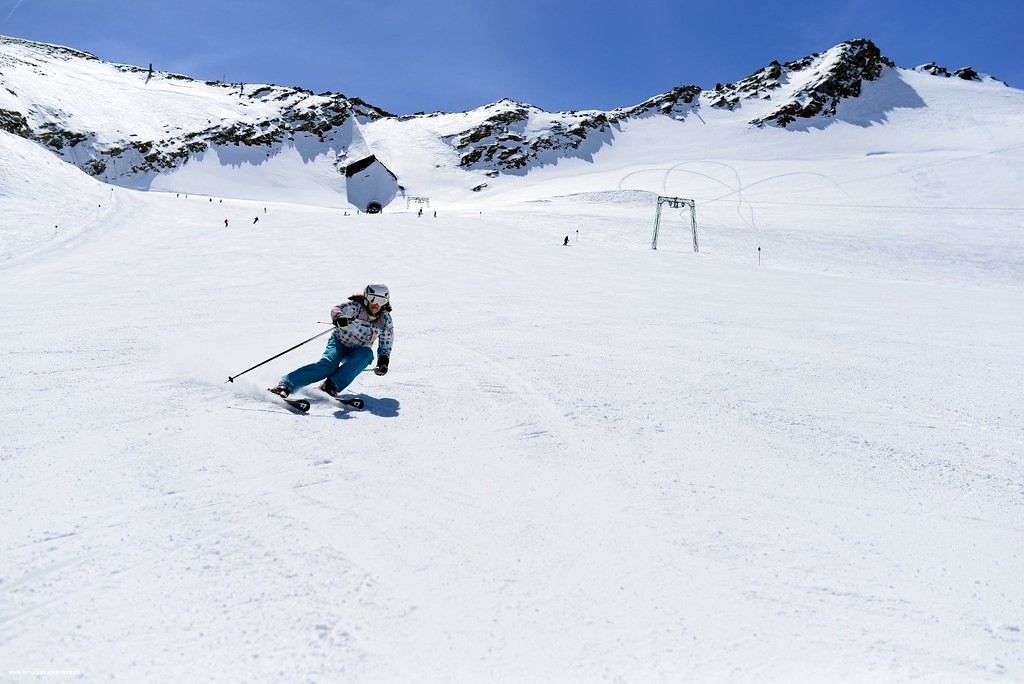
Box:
[283,395,367,413]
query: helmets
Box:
[363,282,390,298]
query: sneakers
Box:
[319,380,337,397]
[273,383,290,398]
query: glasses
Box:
[366,294,389,306]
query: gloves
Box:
[374,365,388,376]
[337,317,351,330]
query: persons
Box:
[253,217,258,223]
[268,284,394,397]
[434,211,436,216]
[225,219,229,226]
[563,236,568,245]
[418,208,422,216]
